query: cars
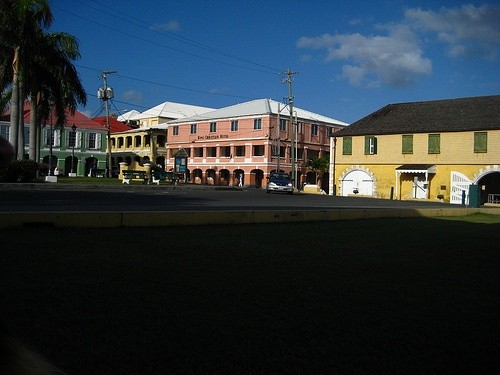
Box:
[267,173,294,193]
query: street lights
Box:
[46,93,55,176]
[70,123,77,173]
